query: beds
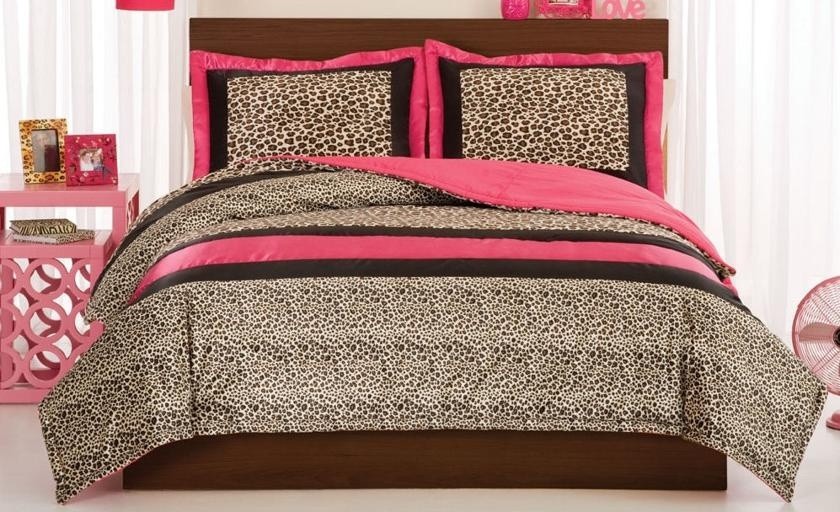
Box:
[37,18,828,503]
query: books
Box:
[14,229,95,243]
[10,219,76,237]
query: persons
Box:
[79,151,103,172]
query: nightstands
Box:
[0,174,141,405]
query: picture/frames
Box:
[64,134,118,186]
[19,119,68,184]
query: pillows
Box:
[424,38,665,201]
[190,47,427,182]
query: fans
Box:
[792,277,840,431]
